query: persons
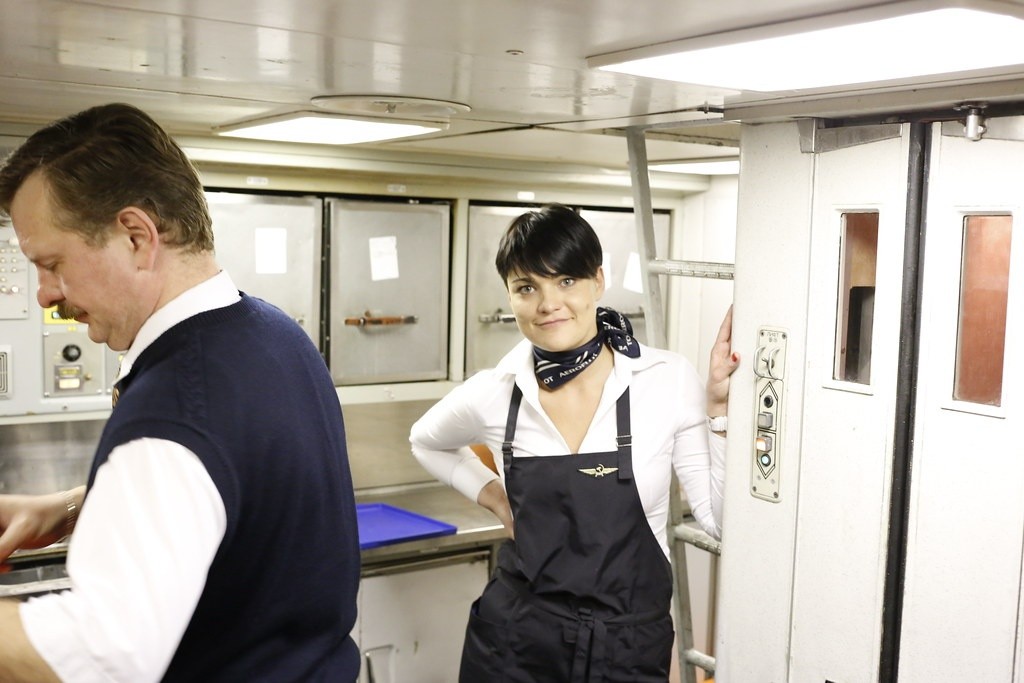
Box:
[409,202,740,683]
[0,102,364,683]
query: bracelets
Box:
[57,488,76,538]
[705,414,727,432]
